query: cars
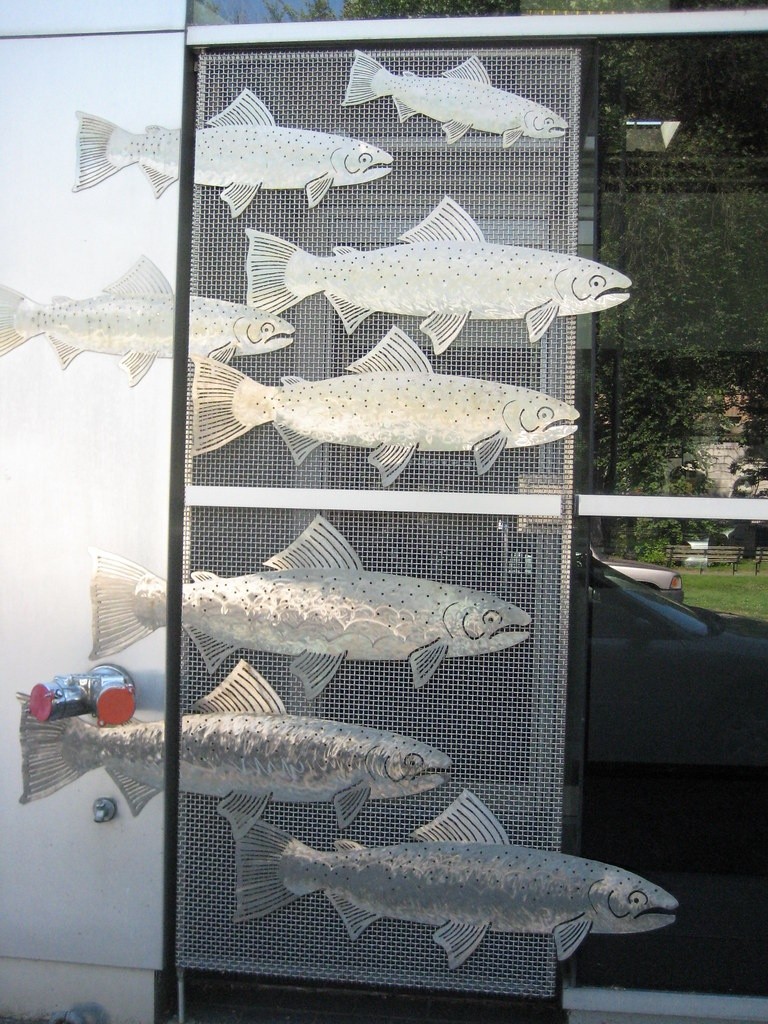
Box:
[586,554,768,876]
[589,543,686,604]
